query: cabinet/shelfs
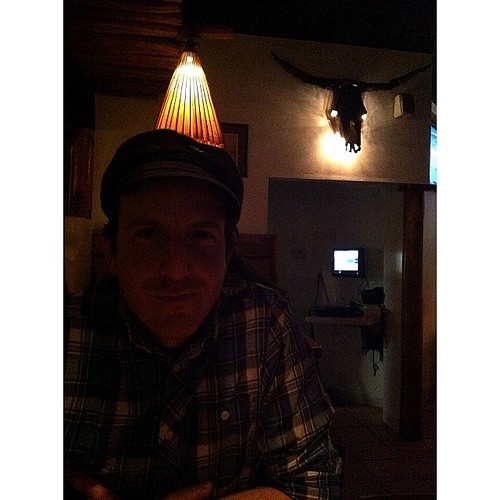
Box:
[89,228,278,287]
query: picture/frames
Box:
[221,123,248,178]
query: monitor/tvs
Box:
[331,247,365,277]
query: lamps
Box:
[152,36,226,150]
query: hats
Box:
[98,128,246,224]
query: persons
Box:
[63,129,344,500]
